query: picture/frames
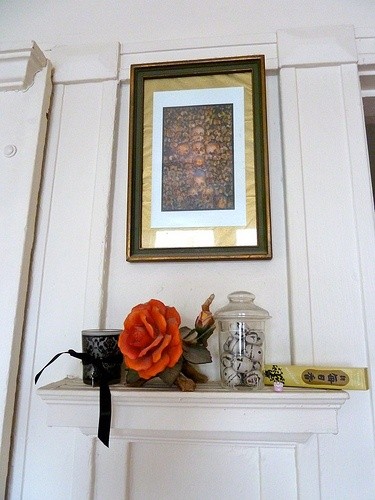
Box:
[125,54,273,262]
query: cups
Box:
[82,329,122,385]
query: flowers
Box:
[119,293,216,392]
